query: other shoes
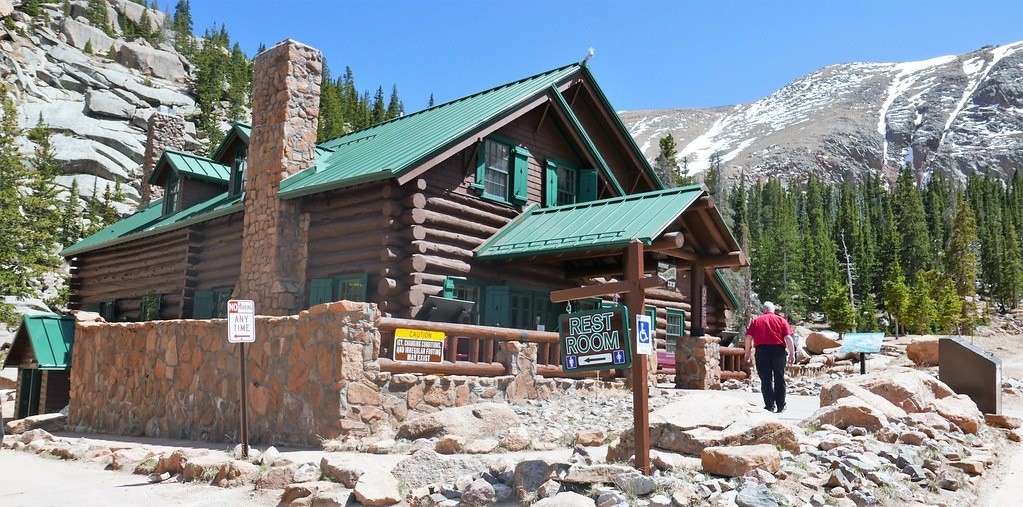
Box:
[764,406,774,412]
[778,404,784,412]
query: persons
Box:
[744,301,795,412]
